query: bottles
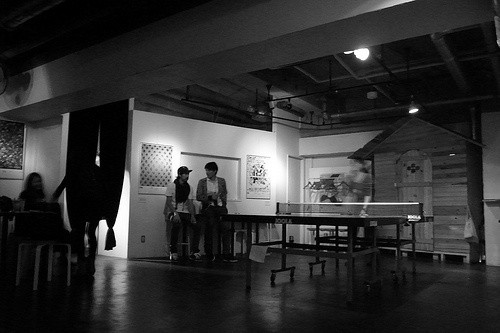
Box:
[213,192,218,205]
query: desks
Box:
[224,201,435,288]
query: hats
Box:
[177,166,193,175]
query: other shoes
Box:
[169,251,178,261]
[189,252,202,261]
[223,257,240,263]
[208,256,216,261]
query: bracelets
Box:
[363,208,366,211]
[174,212,177,216]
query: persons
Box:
[52,163,100,274]
[197,162,239,264]
[14,172,47,238]
[163,166,201,259]
[339,156,380,267]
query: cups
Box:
[12,197,25,211]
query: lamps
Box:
[277,101,292,111]
[408,94,419,114]
[367,88,378,100]
[264,84,275,109]
